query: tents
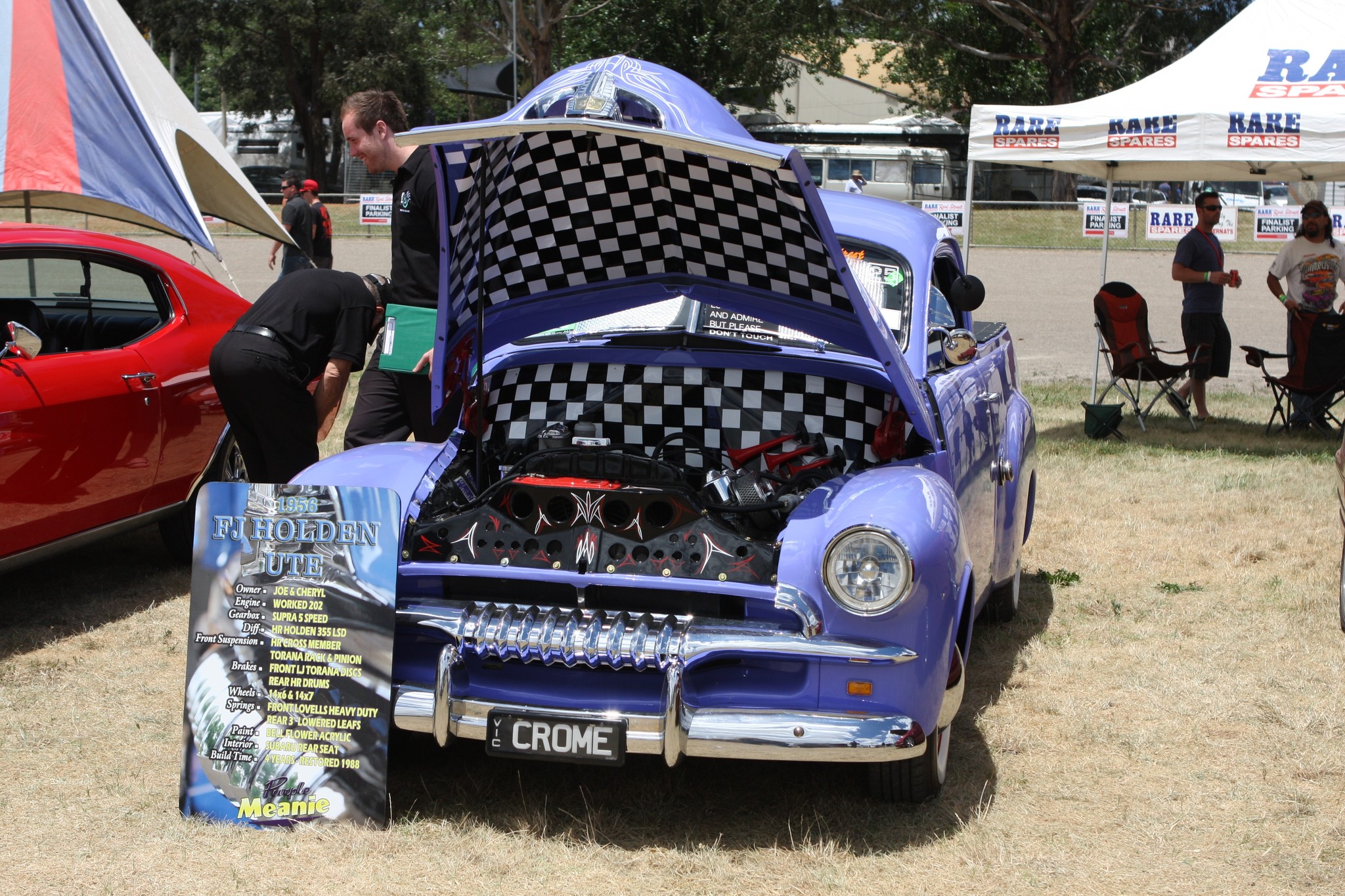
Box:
[961,0,1345,405]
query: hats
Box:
[299,179,318,192]
[365,273,392,346]
[1299,200,1327,211]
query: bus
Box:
[223,119,396,204]
[773,143,953,210]
[736,114,1055,211]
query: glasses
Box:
[1301,212,1326,220]
[1203,205,1222,212]
[279,185,290,190]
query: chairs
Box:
[1239,308,1345,438]
[0,297,50,358]
[1092,281,1213,437]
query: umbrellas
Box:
[0,0,319,299]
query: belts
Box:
[228,323,284,345]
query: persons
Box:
[282,169,297,208]
[844,169,867,194]
[268,172,316,281]
[1158,181,1171,202]
[208,269,393,484]
[1268,200,1345,431]
[1165,191,1242,421]
[1172,182,1182,204]
[341,87,464,451]
[298,179,333,269]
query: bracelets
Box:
[1204,271,1211,283]
[1278,294,1289,306]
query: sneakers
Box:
[1196,414,1217,425]
[1166,391,1190,420]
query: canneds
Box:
[1229,269,1238,287]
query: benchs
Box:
[41,310,161,353]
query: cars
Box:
[282,55,1037,799]
[0,222,249,572]
[1076,173,1290,213]
